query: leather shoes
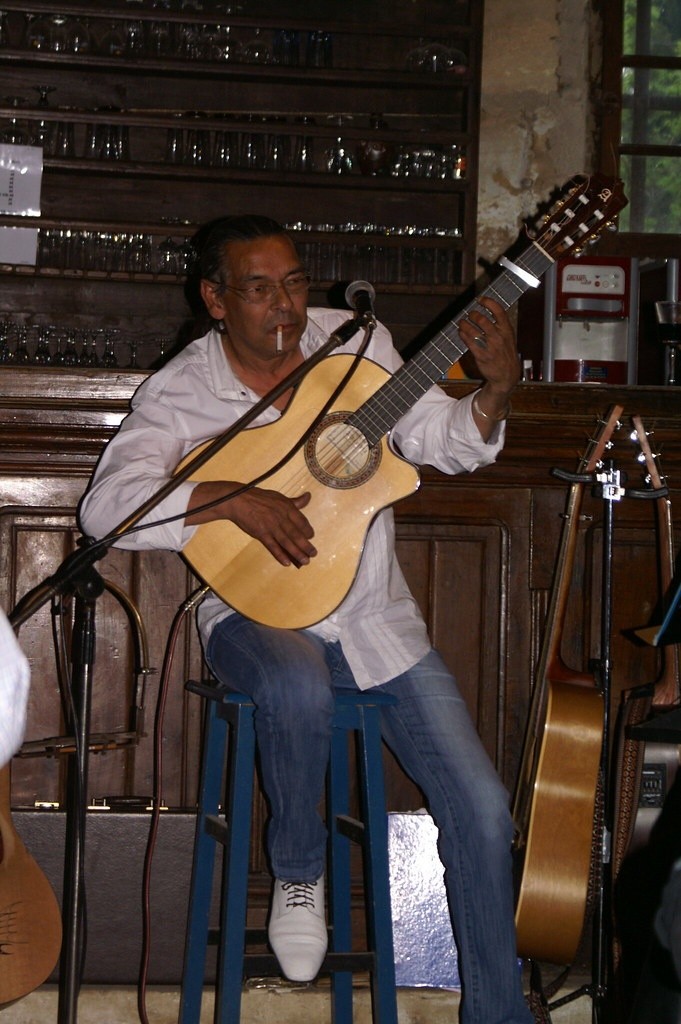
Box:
[268,873,338,981]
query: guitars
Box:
[503,395,681,968]
[168,169,634,625]
[0,762,64,1006]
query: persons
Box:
[77,212,538,1024]
[0,603,64,1006]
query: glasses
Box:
[207,269,314,304]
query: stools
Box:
[175,677,398,1024]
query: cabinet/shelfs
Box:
[0,0,485,379]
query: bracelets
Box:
[473,391,511,421]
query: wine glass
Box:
[240,29,272,63]
[27,86,57,156]
[2,96,26,145]
[327,115,360,175]
[178,216,197,275]
[160,217,177,274]
[0,321,171,368]
[215,5,244,63]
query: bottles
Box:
[167,111,315,174]
[364,113,399,175]
[54,107,130,162]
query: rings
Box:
[473,335,490,349]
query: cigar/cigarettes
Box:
[277,325,283,352]
